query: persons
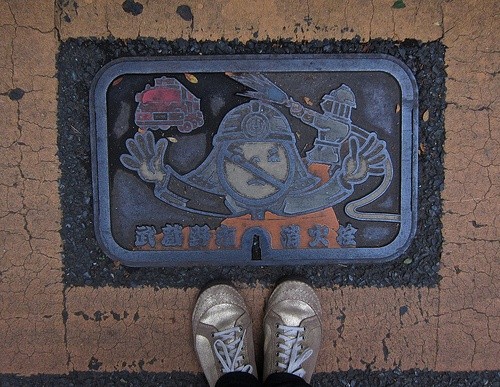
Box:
[190,278,322,387]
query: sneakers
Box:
[191,274,322,387]
